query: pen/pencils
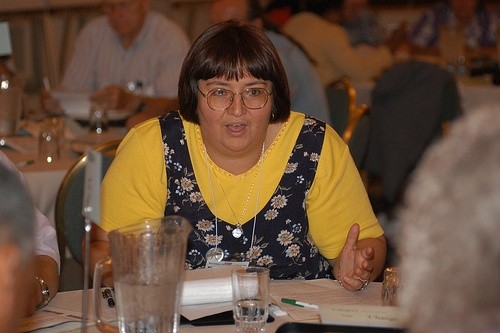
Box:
[101,288,115,307]
[17,160,35,168]
[43,77,51,90]
[281,297,318,309]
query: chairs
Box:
[269,30,333,125]
[342,99,375,191]
[57,135,122,294]
[324,72,358,132]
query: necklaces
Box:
[196,123,266,238]
[203,145,264,261]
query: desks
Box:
[0,123,127,258]
[27,267,408,333]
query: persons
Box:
[80,20,387,291]
[390,103,499,332]
[0,129,61,333]
[0,0,500,222]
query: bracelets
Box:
[338,261,344,288]
[134,96,146,115]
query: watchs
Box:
[30,271,51,312]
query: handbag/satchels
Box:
[326,78,351,135]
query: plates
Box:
[71,112,128,122]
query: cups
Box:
[89,103,109,138]
[382,267,401,306]
[37,118,64,170]
[231,267,270,333]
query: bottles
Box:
[0,54,18,136]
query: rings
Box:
[356,276,368,291]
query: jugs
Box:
[92,214,193,333]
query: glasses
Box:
[197,85,272,111]
